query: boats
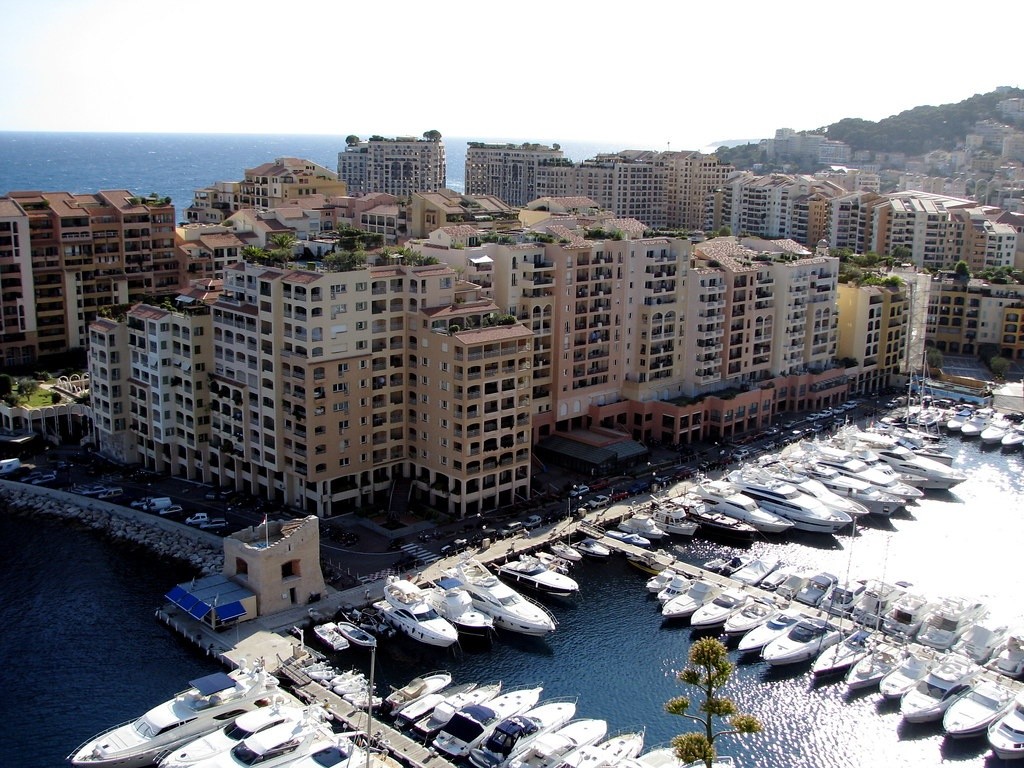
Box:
[142,391,1024,768]
[67,655,293,768]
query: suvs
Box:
[522,515,541,529]
[1002,412,1024,422]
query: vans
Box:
[142,496,171,512]
[0,458,20,475]
[497,521,523,537]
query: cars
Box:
[200,518,229,530]
[82,486,108,496]
[570,483,589,496]
[32,474,56,486]
[20,471,43,482]
[5,466,31,481]
[591,477,611,491]
[440,538,467,555]
[131,497,154,509]
[99,487,124,501]
[160,504,184,516]
[545,508,565,524]
[185,512,208,526]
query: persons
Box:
[509,541,515,553]
[364,589,370,600]
